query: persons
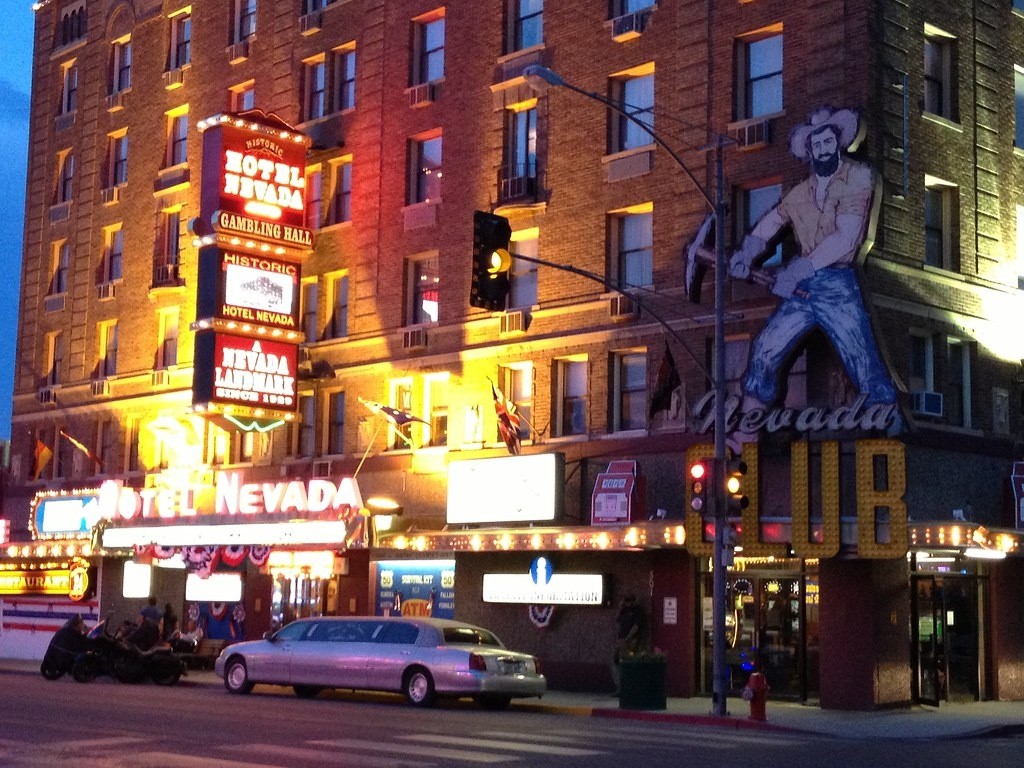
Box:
[720,105,911,442]
[179,620,205,653]
[160,602,180,649]
[137,595,165,641]
[609,589,648,700]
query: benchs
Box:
[179,639,226,672]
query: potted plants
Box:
[617,648,668,712]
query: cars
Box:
[214,613,547,712]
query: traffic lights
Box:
[720,454,750,518]
[690,459,708,515]
[469,210,513,312]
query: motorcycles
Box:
[42,617,103,681]
[73,616,197,686]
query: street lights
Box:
[522,64,731,719]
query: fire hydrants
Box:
[743,671,771,722]
